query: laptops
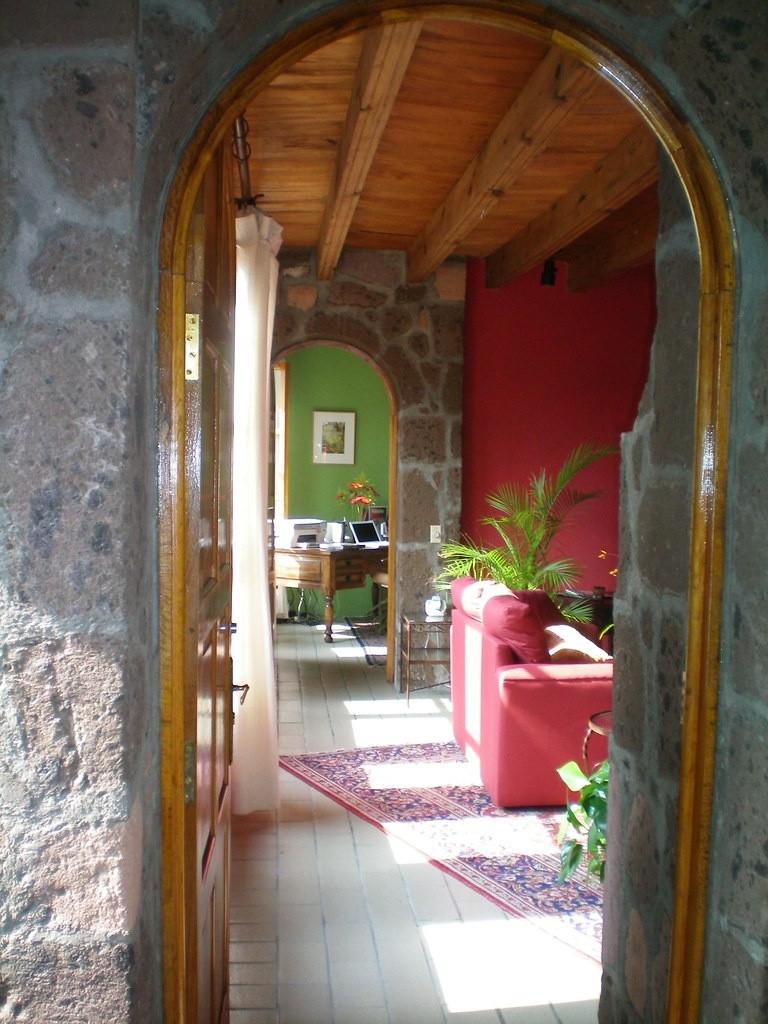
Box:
[349,521,389,548]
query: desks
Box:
[273,542,388,643]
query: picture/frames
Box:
[311,410,356,466]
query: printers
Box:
[273,518,327,548]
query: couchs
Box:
[447,577,614,807]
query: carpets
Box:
[344,616,388,668]
[278,743,604,964]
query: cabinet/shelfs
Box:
[399,614,452,708]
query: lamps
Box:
[540,259,556,286]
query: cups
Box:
[332,523,344,543]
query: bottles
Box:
[425,595,447,617]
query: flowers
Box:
[335,480,380,521]
